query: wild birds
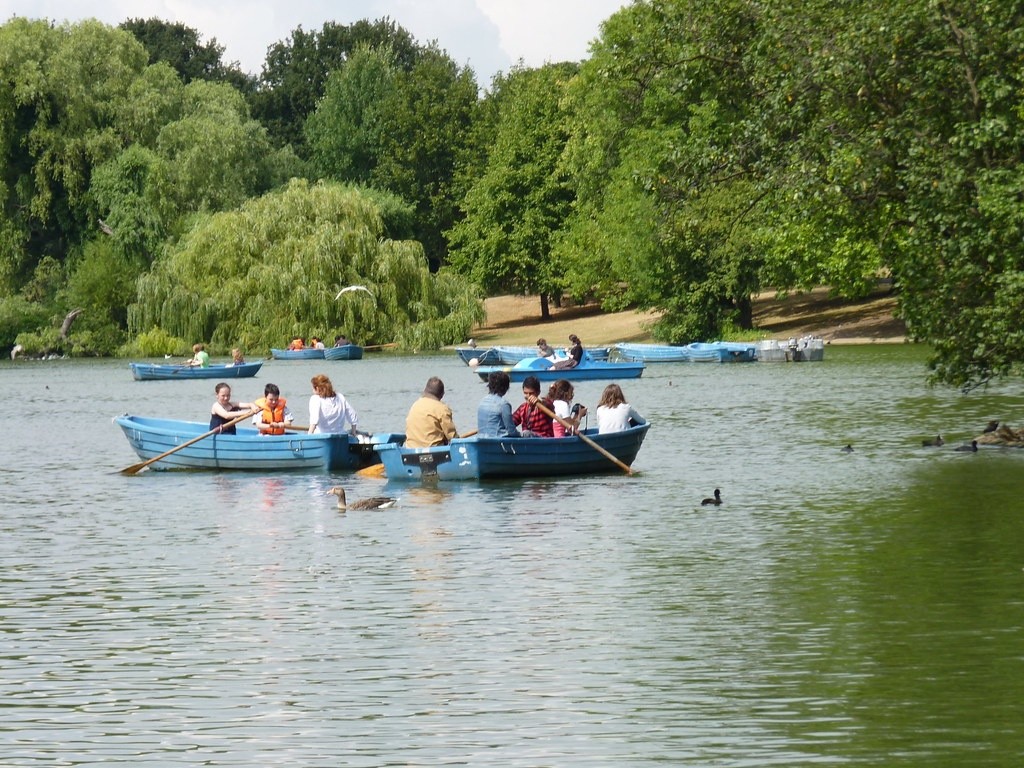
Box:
[840,443,855,453]
[922,435,945,446]
[700,488,723,505]
[955,440,977,452]
[982,419,999,433]
[668,380,672,386]
[327,487,399,511]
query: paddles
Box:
[266,347,289,360]
[269,423,372,437]
[363,343,397,348]
[118,406,264,474]
[525,394,640,478]
[357,429,478,478]
[171,364,192,374]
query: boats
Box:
[454,347,502,365]
[617,342,689,363]
[270,348,327,359]
[686,343,758,363]
[129,360,264,380]
[472,351,647,383]
[373,416,652,482]
[324,345,363,361]
[490,346,609,365]
[115,413,406,471]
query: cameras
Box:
[572,403,586,416]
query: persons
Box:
[536,339,561,360]
[547,379,588,437]
[184,335,350,369]
[596,383,646,435]
[252,383,294,435]
[477,371,522,437]
[510,377,555,438]
[404,377,460,448]
[307,374,358,436]
[549,334,583,370]
[209,383,260,434]
